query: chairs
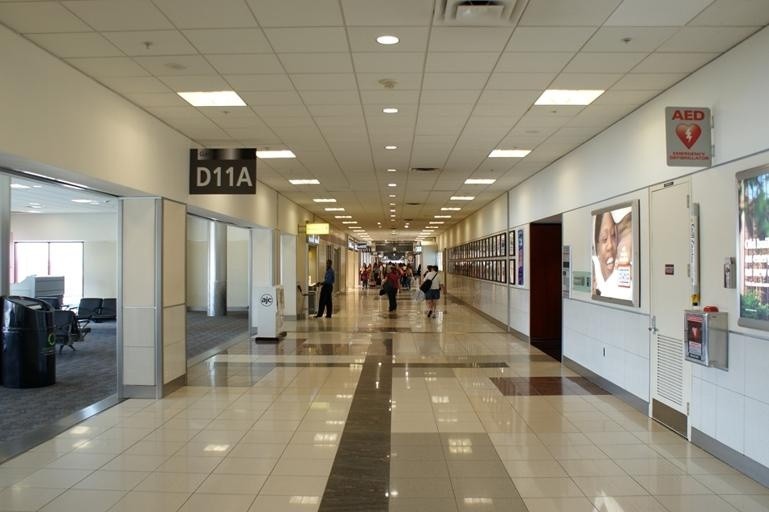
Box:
[40,297,116,355]
[297,284,317,321]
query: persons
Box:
[591,212,633,296]
[313,259,335,318]
[360,261,443,318]
[601,207,634,296]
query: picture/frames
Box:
[446,229,515,285]
[735,162,768,332]
[589,199,641,308]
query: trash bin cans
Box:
[0,296,56,389]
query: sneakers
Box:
[427,310,436,318]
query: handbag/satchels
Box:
[379,284,386,296]
[419,272,437,293]
